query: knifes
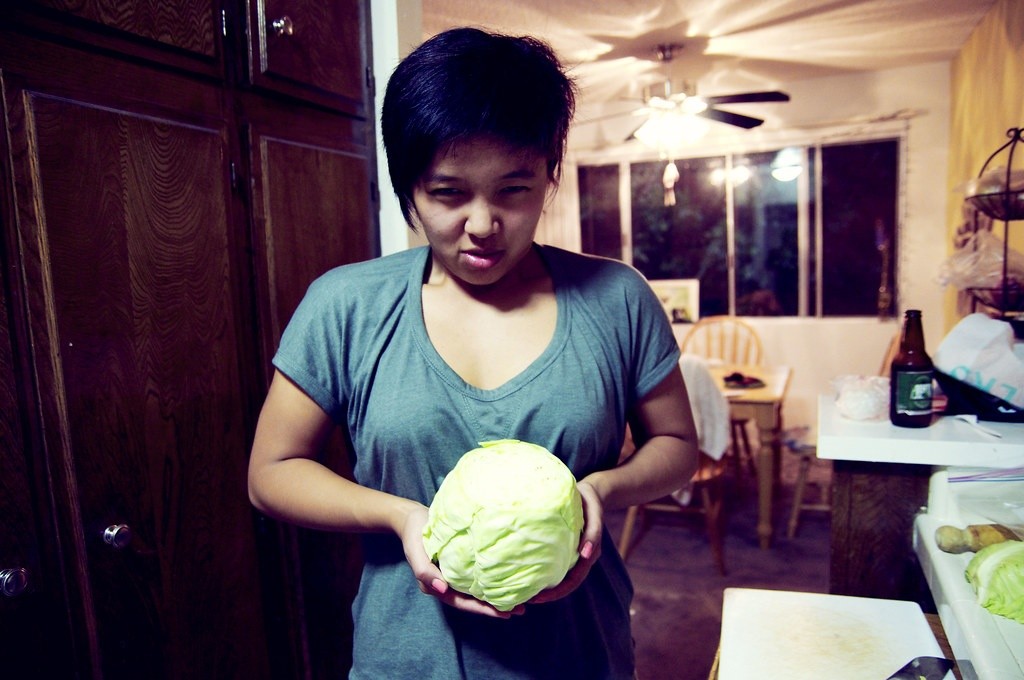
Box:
[886,656,955,680]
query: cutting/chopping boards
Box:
[717,586,957,680]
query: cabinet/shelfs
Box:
[0,0,382,679]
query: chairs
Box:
[786,315,900,542]
[681,316,764,477]
[618,355,732,571]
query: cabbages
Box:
[965,540,1024,625]
[423,437,585,612]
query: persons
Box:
[246,27,700,680]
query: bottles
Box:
[888,309,934,429]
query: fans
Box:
[598,84,791,147]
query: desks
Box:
[703,364,794,549]
[816,394,1024,614]
